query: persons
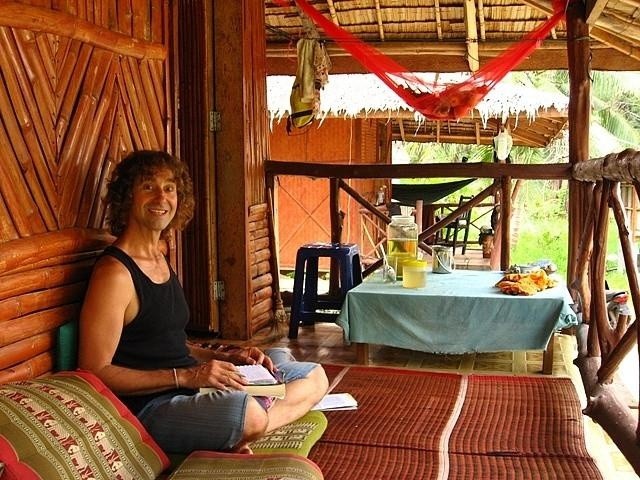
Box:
[75,148,330,456]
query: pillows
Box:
[169,447,325,480]
[1,372,169,477]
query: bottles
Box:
[386,215,419,282]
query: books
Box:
[198,360,286,401]
[310,392,358,412]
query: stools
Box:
[287,240,364,339]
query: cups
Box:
[402,259,429,288]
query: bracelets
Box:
[173,367,181,391]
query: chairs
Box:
[434,195,474,256]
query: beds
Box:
[0,226,605,480]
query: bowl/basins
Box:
[519,264,540,274]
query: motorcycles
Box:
[605,267,634,332]
